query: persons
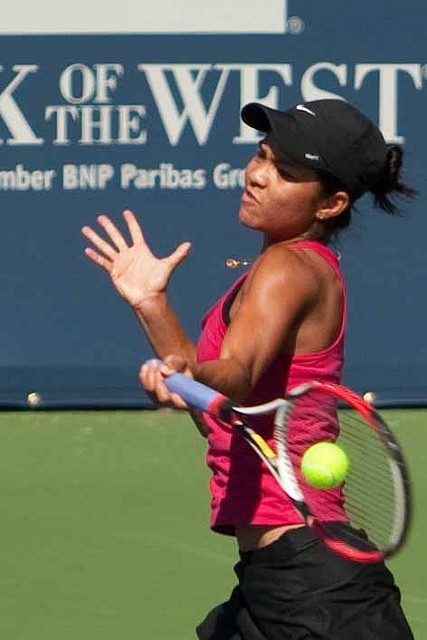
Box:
[78,97,413,640]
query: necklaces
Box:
[227,254,256,270]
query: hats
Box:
[240,98,389,194]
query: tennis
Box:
[301,441,350,490]
